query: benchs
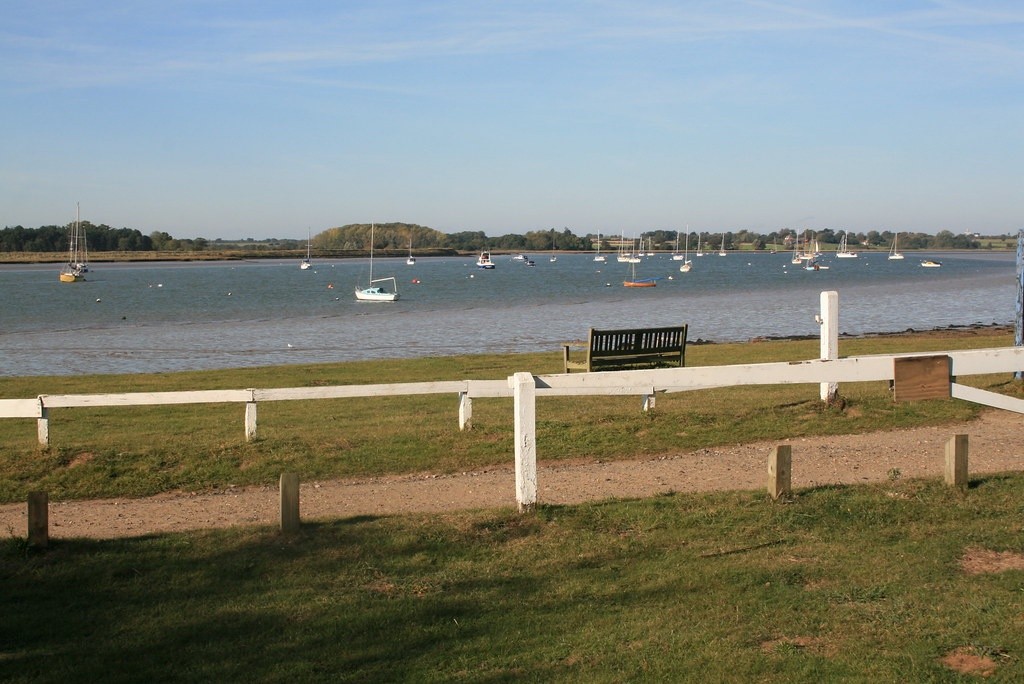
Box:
[561,323,688,373]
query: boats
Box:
[550,254,557,263]
[524,260,536,267]
[475,247,495,269]
[513,254,528,261]
[803,266,832,272]
[300,257,313,270]
[920,258,944,268]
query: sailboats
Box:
[354,218,400,302]
[672,227,683,261]
[404,238,417,265]
[593,226,607,262]
[791,224,904,265]
[616,229,630,262]
[623,237,657,287]
[696,229,704,257]
[680,224,692,272]
[628,231,654,264]
[719,230,727,257]
[59,200,89,282]
